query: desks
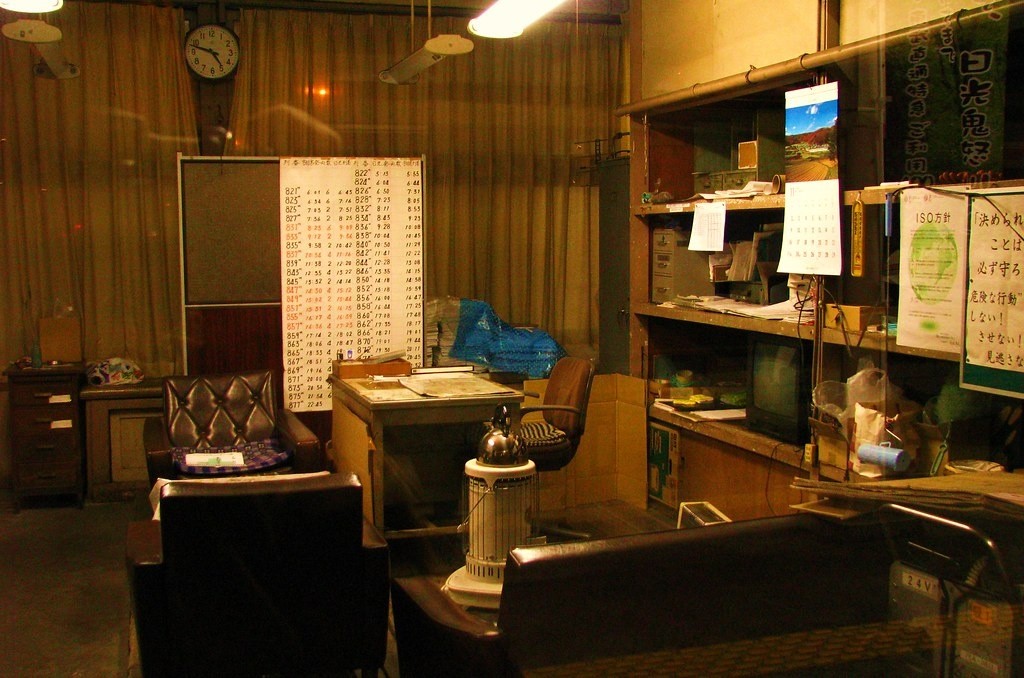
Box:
[330,357,525,554]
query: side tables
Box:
[3,361,85,519]
[79,378,164,503]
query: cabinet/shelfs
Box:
[627,68,1024,613]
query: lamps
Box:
[468,0,568,39]
[379,0,474,86]
[0,17,81,79]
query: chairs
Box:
[457,355,603,541]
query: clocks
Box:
[184,0,242,156]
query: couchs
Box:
[145,370,320,515]
[125,472,389,678]
[392,507,890,678]
